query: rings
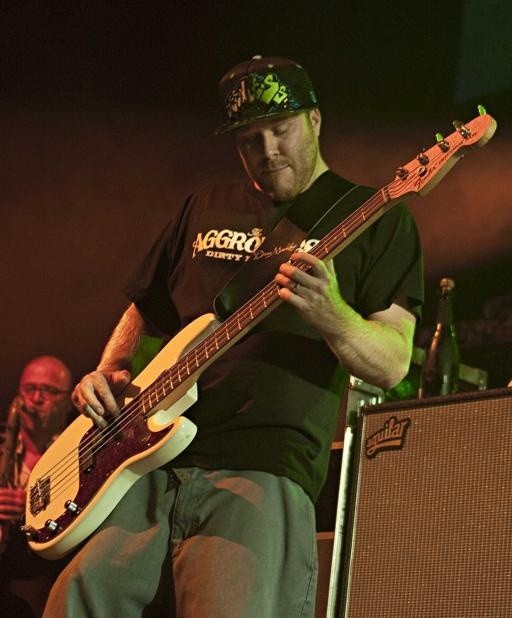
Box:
[292,282,300,293]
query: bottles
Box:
[419,276,461,398]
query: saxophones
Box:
[0,395,38,556]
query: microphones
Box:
[426,277,456,352]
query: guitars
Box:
[20,105,497,562]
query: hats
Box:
[214,54,318,135]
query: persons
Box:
[2,352,73,618]
[35,57,424,617]
